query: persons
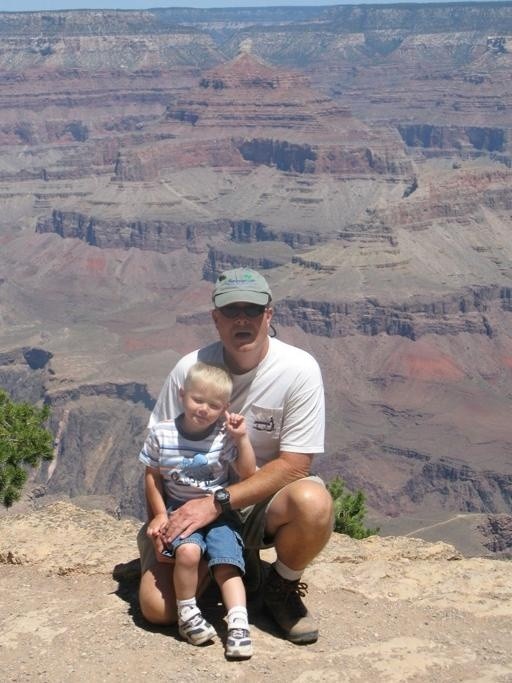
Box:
[137,362,256,659]
[133,266,336,644]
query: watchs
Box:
[215,488,233,514]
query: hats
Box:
[212,267,272,308]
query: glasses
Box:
[220,305,265,320]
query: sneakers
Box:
[247,551,261,592]
[178,604,217,646]
[261,563,318,644]
[225,618,253,658]
